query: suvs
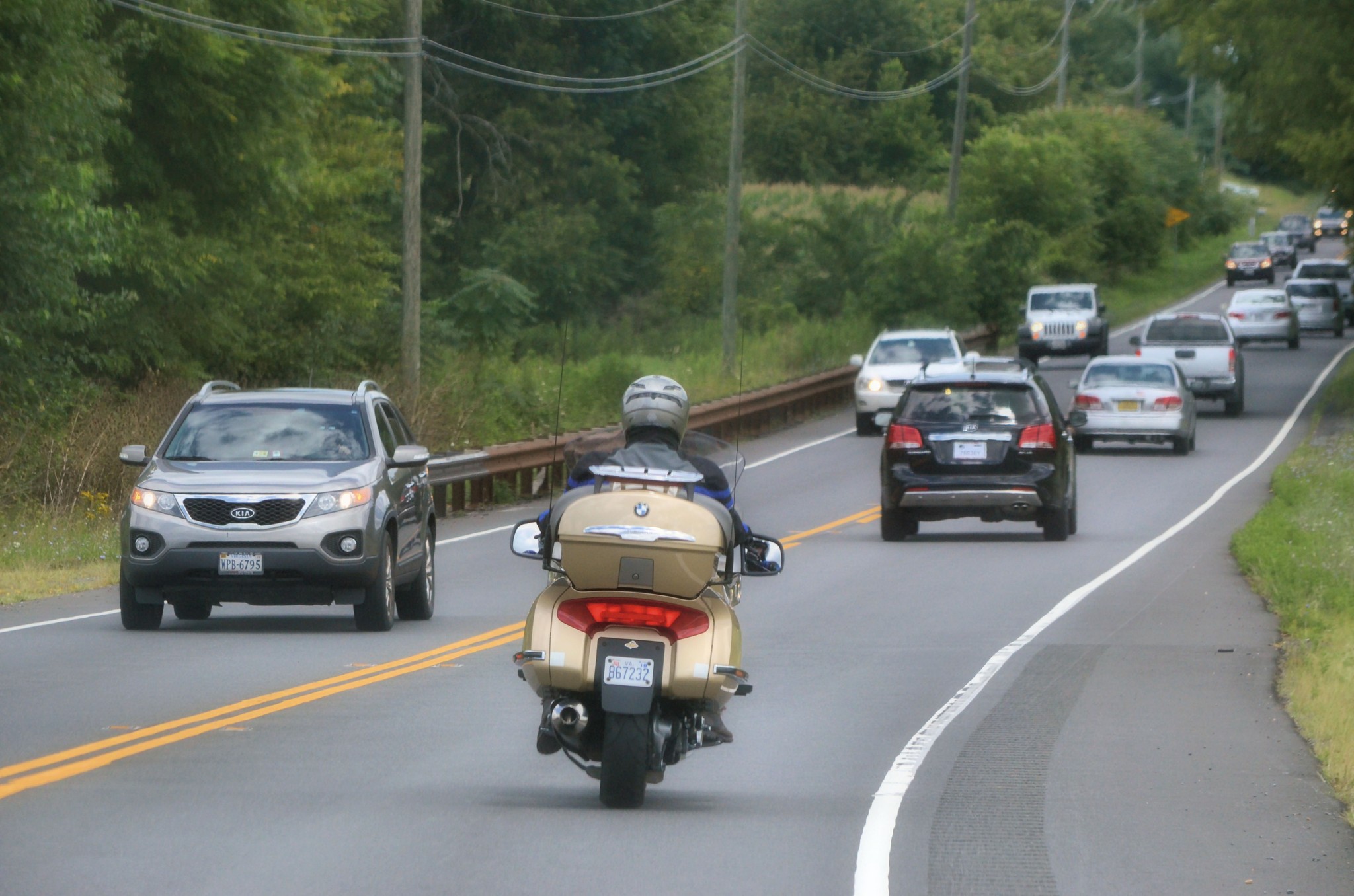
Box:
[872,357,1088,541]
[1286,258,1354,329]
[1277,215,1316,252]
[119,379,436,632]
[1221,241,1276,288]
[1016,282,1111,368]
[849,326,979,435]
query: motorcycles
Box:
[510,431,785,805]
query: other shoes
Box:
[537,697,563,754]
[699,710,734,744]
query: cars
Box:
[1314,205,1350,238]
[1225,289,1300,350]
[1256,230,1299,268]
[1130,312,1245,414]
[1283,277,1348,336]
[1065,354,1197,455]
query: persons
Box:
[323,416,362,459]
[536,375,753,755]
[747,537,780,571]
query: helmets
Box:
[621,375,690,447]
[745,536,770,560]
